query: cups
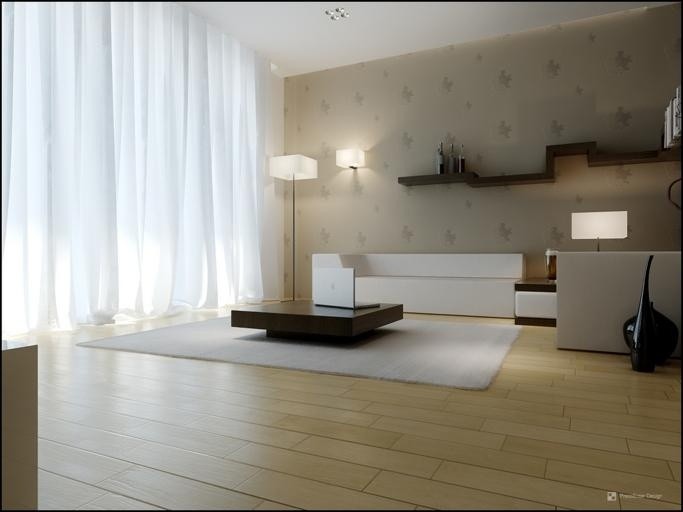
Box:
[544,248,561,282]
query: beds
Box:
[230,298,402,343]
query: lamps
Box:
[265,156,317,304]
[571,211,627,250]
[335,149,364,170]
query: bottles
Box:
[630,256,659,372]
[664,87,681,148]
[436,142,465,174]
[623,301,679,366]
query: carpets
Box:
[74,312,524,396]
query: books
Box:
[662,84,680,149]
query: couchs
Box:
[309,246,527,319]
